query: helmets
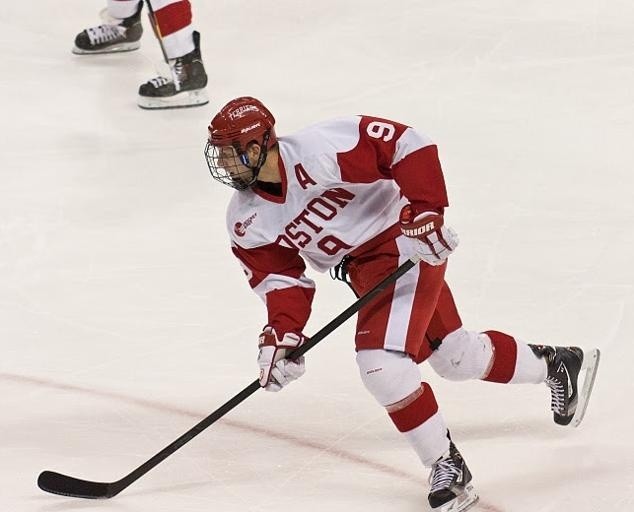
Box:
[204,96,277,191]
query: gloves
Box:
[257,325,310,392]
[398,204,458,267]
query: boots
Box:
[140,29,207,97]
[527,343,584,426]
[75,0,143,50]
[428,428,471,509]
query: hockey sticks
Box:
[145,1,180,89]
[38,253,419,501]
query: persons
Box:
[199,93,584,508]
[74,0,208,100]
